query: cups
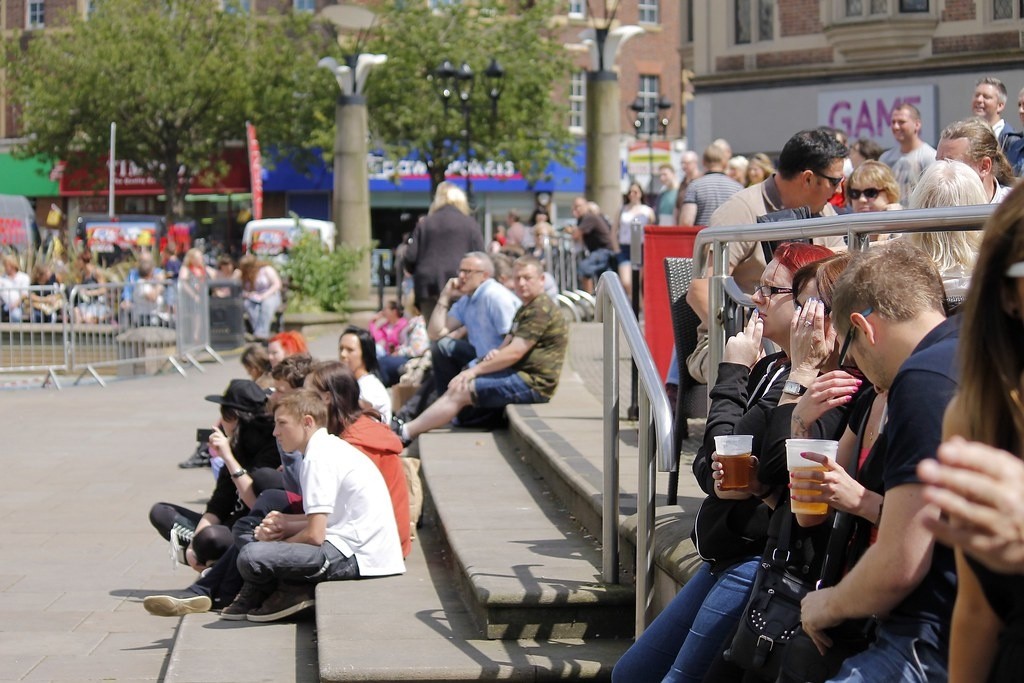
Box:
[785,438,839,514]
[713,434,755,490]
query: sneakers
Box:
[222,579,315,623]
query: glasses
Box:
[752,284,794,298]
[838,309,874,378]
[455,268,485,275]
[847,188,888,198]
[802,169,845,186]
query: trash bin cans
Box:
[209,278,246,349]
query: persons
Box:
[610,179,1024,683]
[393,253,569,447]
[369,181,486,387]
[485,76,1024,297]
[220,388,406,624]
[392,251,524,433]
[0,238,284,341]
[143,361,411,616]
[149,324,393,561]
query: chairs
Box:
[663,257,708,506]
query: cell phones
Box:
[197,428,214,441]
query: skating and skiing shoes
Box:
[143,587,210,616]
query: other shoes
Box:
[166,522,196,566]
[391,411,412,448]
[179,451,212,469]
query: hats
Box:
[207,378,269,413]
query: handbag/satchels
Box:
[725,558,872,682]
